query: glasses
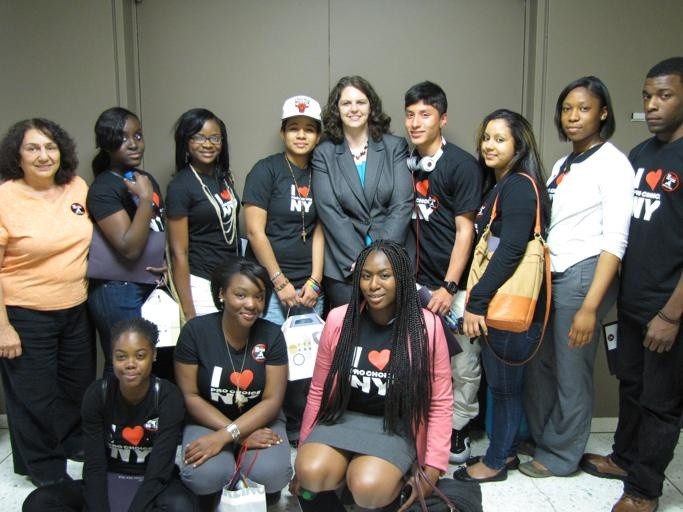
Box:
[190,135,221,145]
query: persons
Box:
[580,53,682,512]
[22,316,200,511]
[241,93,329,332]
[1,114,95,494]
[517,75,637,477]
[164,109,240,334]
[83,106,168,385]
[173,256,294,512]
[404,80,479,465]
[288,239,456,511]
[312,75,416,319]
[451,106,553,483]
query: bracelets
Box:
[225,422,243,443]
[308,276,322,290]
[306,278,321,296]
[273,277,289,294]
[269,270,284,282]
[654,310,682,329]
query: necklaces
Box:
[188,160,239,246]
[348,138,369,161]
[219,326,249,409]
[563,138,605,174]
[284,150,313,242]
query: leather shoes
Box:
[465,455,519,470]
[29,472,73,488]
[579,452,628,479]
[611,491,659,512]
[452,464,508,482]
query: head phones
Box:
[406,137,448,173]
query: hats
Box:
[280,95,322,123]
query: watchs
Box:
[439,281,460,296]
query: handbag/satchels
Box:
[139,274,181,348]
[396,465,483,512]
[280,296,325,382]
[464,233,545,334]
[206,441,268,512]
[84,220,167,285]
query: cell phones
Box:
[457,318,466,336]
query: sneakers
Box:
[518,439,534,456]
[449,424,473,464]
[517,460,558,477]
